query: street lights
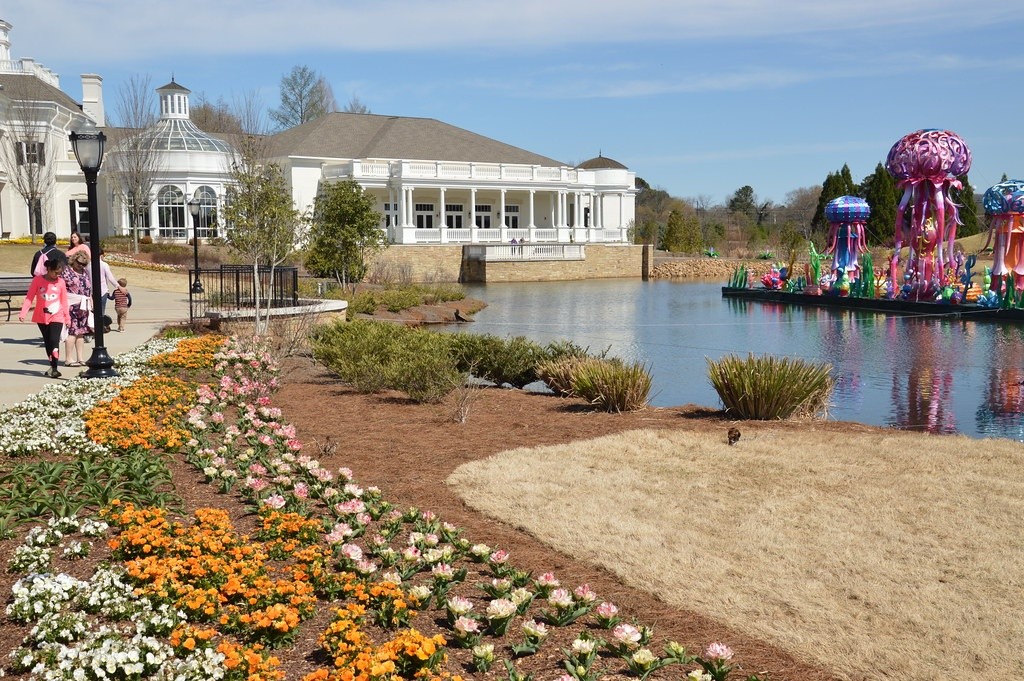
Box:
[188,196,206,294]
[67,118,124,379]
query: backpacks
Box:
[33,248,55,276]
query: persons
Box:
[18,232,132,378]
[511,237,525,256]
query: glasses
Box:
[76,259,87,267]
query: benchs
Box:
[0,277,33,323]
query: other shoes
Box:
[64,362,81,366]
[77,361,87,366]
[118,329,120,331]
[45,367,53,375]
[120,329,126,332]
[51,371,62,378]
